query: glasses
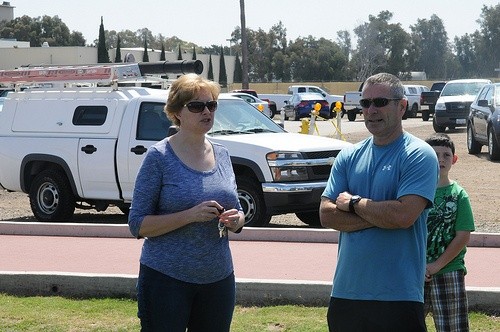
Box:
[183,101,218,113]
[359,97,402,108]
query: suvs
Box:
[432,78,493,133]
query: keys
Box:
[217,222,226,239]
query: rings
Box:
[233,220,238,225]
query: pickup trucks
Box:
[0,77,358,226]
[257,85,346,120]
[420,81,448,122]
[343,84,431,121]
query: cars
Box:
[281,92,330,121]
[466,83,500,161]
[224,90,275,120]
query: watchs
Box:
[349,194,361,215]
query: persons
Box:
[128,74,244,332]
[425,133,474,332]
[318,72,439,332]
